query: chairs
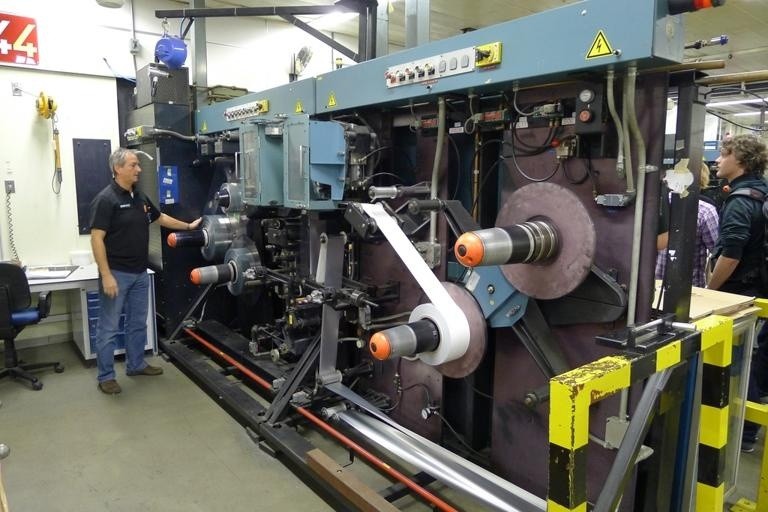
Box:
[1,262,64,390]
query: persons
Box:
[654,182,673,254]
[86,147,204,395]
[705,132,768,455]
[651,154,720,290]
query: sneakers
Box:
[127,365,162,374]
[741,433,758,452]
[101,380,121,393]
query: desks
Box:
[24,264,158,368]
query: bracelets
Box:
[187,222,191,231]
